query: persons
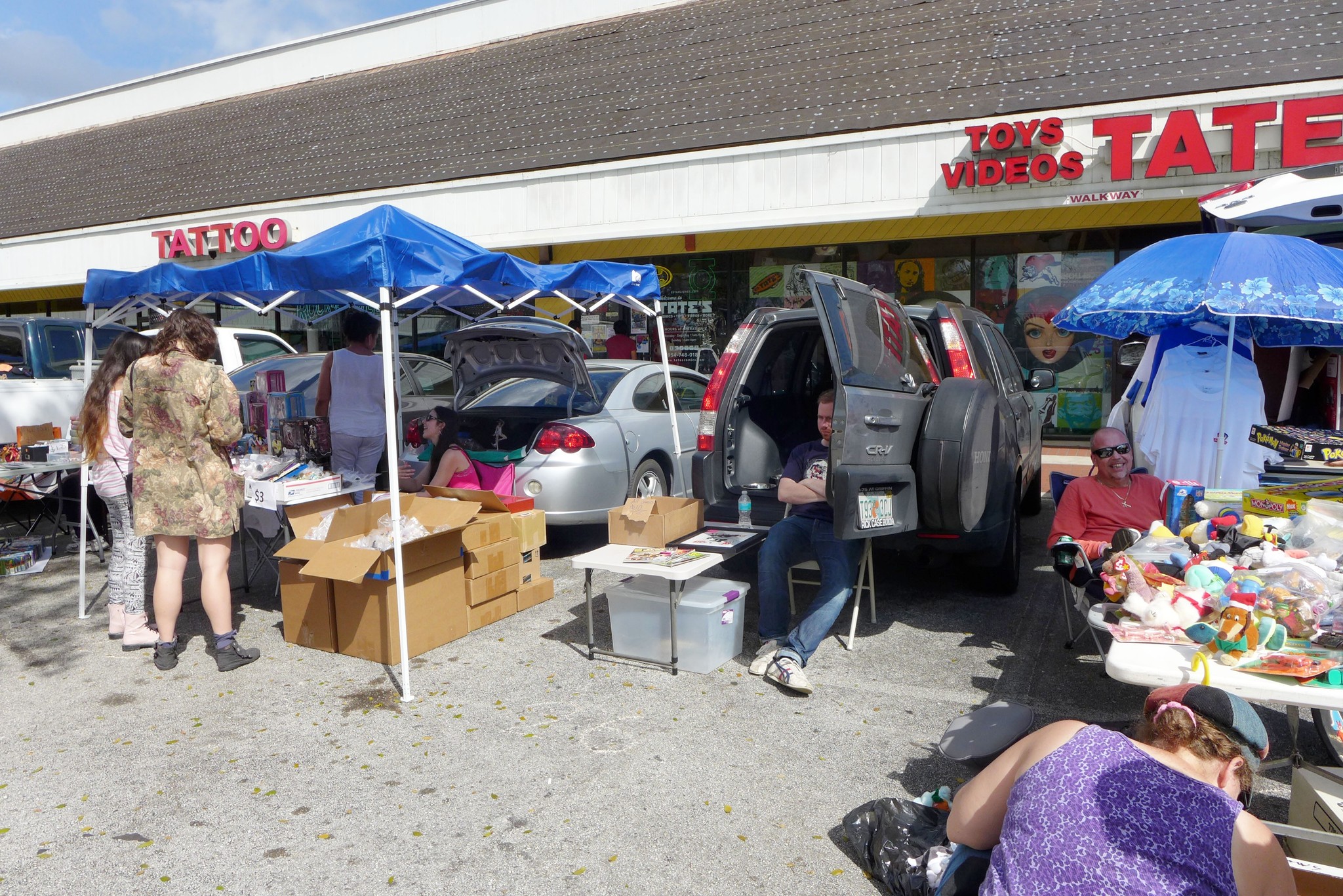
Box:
[944,681,1299,895]
[116,306,262,672]
[1047,427,1168,607]
[564,319,587,363]
[748,390,858,695]
[397,405,480,499]
[492,418,507,451]
[315,311,399,506]
[603,321,638,359]
[75,330,180,653]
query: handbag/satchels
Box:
[124,472,133,510]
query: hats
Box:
[1145,683,1269,760]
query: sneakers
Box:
[1103,528,1142,563]
[66,534,109,553]
[215,639,261,671]
[748,641,782,674]
[153,642,179,670]
[766,658,814,693]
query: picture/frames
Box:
[665,526,770,554]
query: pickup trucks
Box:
[0,317,138,380]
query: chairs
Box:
[1050,460,1169,689]
[781,501,877,651]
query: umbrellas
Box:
[1050,227,1343,488]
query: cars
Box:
[227,351,455,459]
[432,317,713,528]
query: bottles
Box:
[738,490,752,529]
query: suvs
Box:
[692,268,1054,597]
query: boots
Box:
[108,602,127,639]
[121,608,177,651]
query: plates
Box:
[741,483,777,490]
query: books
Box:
[68,415,85,453]
[258,459,301,482]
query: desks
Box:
[236,476,382,596]
[2,455,110,568]
[572,520,776,676]
[1101,609,1343,759]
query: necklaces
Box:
[1096,475,1133,508]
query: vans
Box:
[137,327,299,376]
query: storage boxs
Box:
[277,481,555,668]
[604,574,754,675]
[608,495,708,550]
[1279,762,1343,874]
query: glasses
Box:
[425,414,443,423]
[1093,443,1131,459]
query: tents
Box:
[82,204,688,703]
